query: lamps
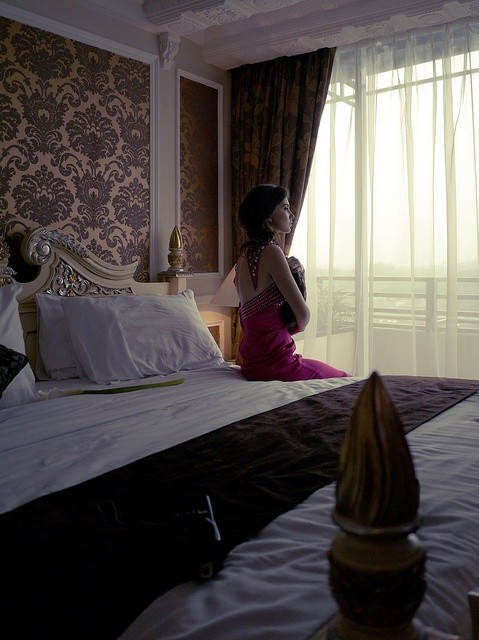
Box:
[210,262,241,364]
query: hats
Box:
[238,186,287,239]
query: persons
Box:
[232,184,352,381]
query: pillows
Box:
[1,281,38,410]
[59,289,227,385]
[0,343,30,400]
[35,289,83,382]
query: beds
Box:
[0,224,478,637]
